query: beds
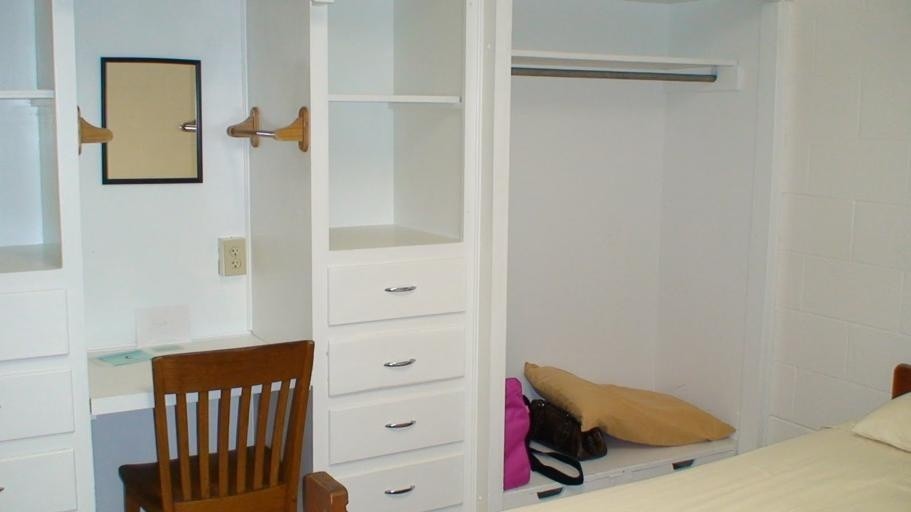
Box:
[513,363,911,511]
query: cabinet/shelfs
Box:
[309,2,485,509]
[505,0,777,499]
[0,0,98,512]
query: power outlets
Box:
[217,236,247,278]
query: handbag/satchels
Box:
[522,394,608,462]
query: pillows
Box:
[523,354,739,451]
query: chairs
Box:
[119,337,353,512]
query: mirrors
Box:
[101,58,204,185]
